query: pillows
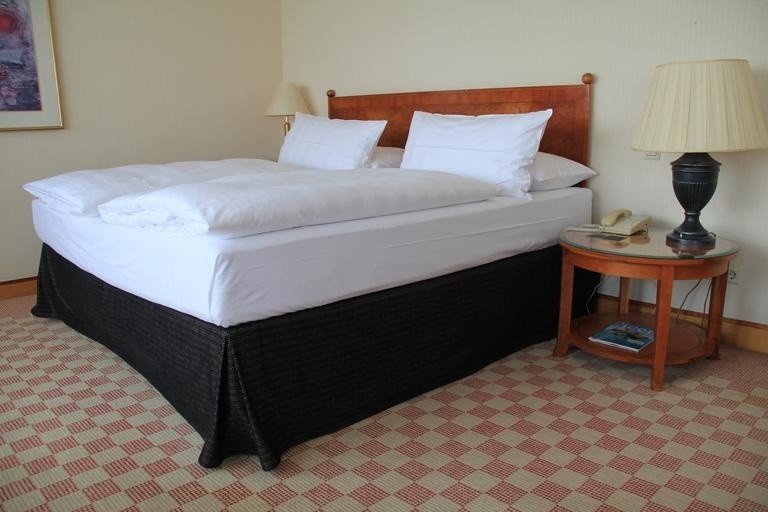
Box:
[277,110,389,171]
[530,151,598,190]
[400,108,554,203]
[372,145,405,170]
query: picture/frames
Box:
[0,0,64,131]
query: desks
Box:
[552,228,741,392]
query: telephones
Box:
[600,208,653,236]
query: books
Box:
[588,320,655,352]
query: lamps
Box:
[631,59,768,253]
[263,82,310,136]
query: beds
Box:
[22,73,602,470]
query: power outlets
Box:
[727,267,741,284]
